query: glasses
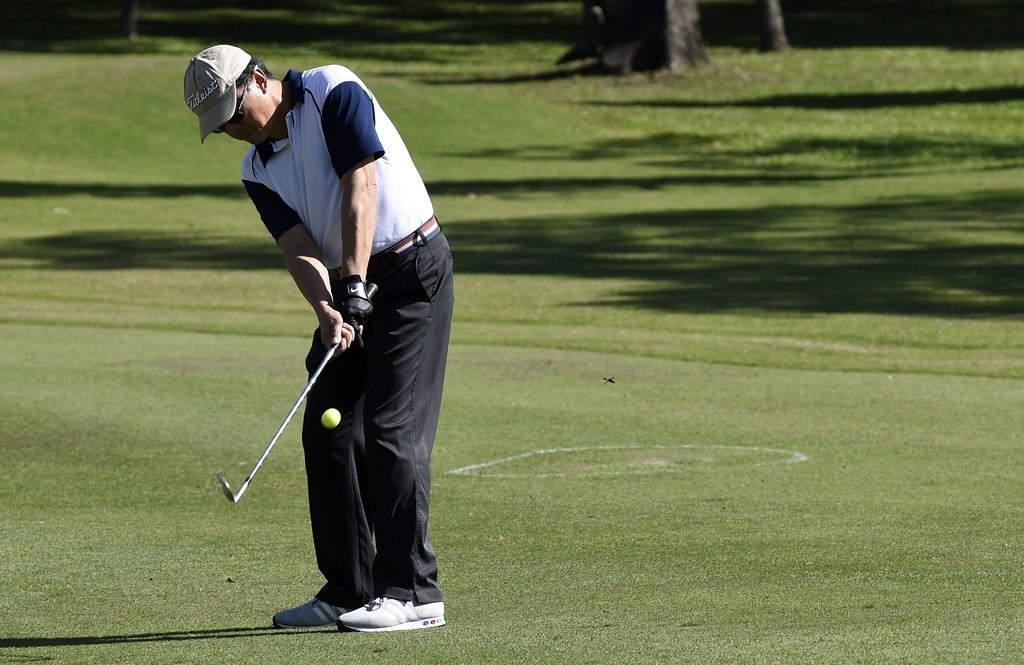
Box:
[210,67,252,135]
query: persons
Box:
[184,45,454,631]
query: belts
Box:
[328,214,441,283]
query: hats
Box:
[183,44,252,144]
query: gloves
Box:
[330,274,375,349]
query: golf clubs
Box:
[216,281,379,504]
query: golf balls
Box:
[322,408,342,429]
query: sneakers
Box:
[336,597,446,632]
[272,596,355,629]
